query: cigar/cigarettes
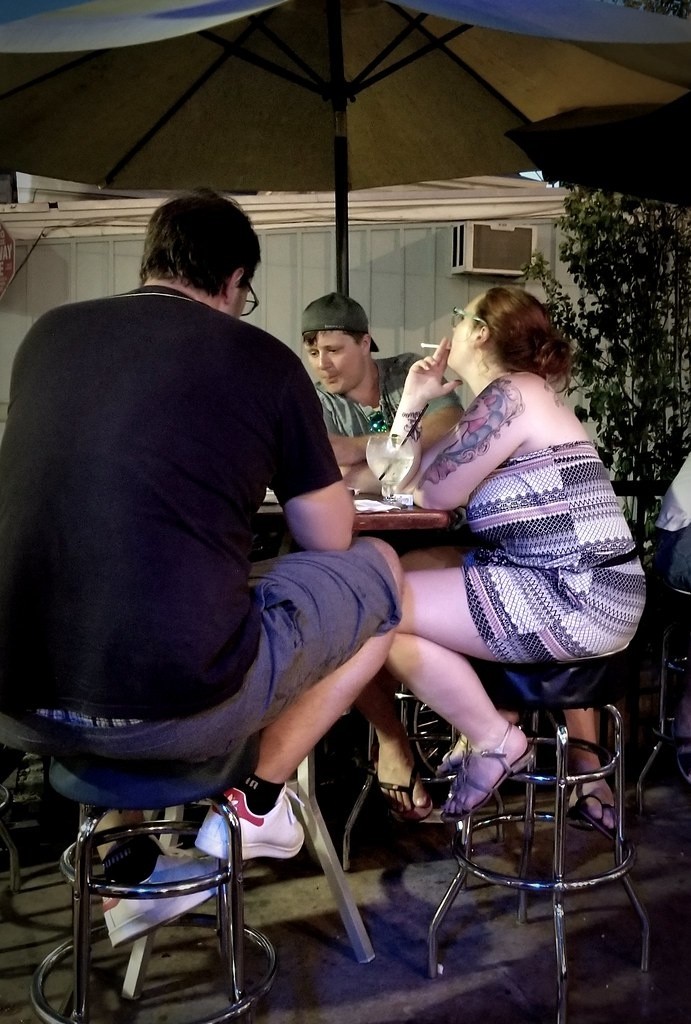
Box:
[421,342,451,349]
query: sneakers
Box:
[102,834,220,948]
[194,782,305,859]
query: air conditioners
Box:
[449,219,539,280]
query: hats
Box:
[301,292,379,352]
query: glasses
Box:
[239,275,259,317]
[451,306,493,337]
[368,411,388,434]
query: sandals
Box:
[440,720,536,823]
[435,732,471,779]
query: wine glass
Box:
[366,434,415,508]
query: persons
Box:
[387,287,645,822]
[0,194,404,949]
[298,291,617,844]
[654,453,691,755]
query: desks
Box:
[114,486,460,1005]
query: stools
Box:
[426,648,652,1024]
[636,580,691,815]
[28,745,278,1024]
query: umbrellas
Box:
[0,0,691,297]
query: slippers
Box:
[671,717,691,784]
[370,741,433,823]
[569,794,616,842]
